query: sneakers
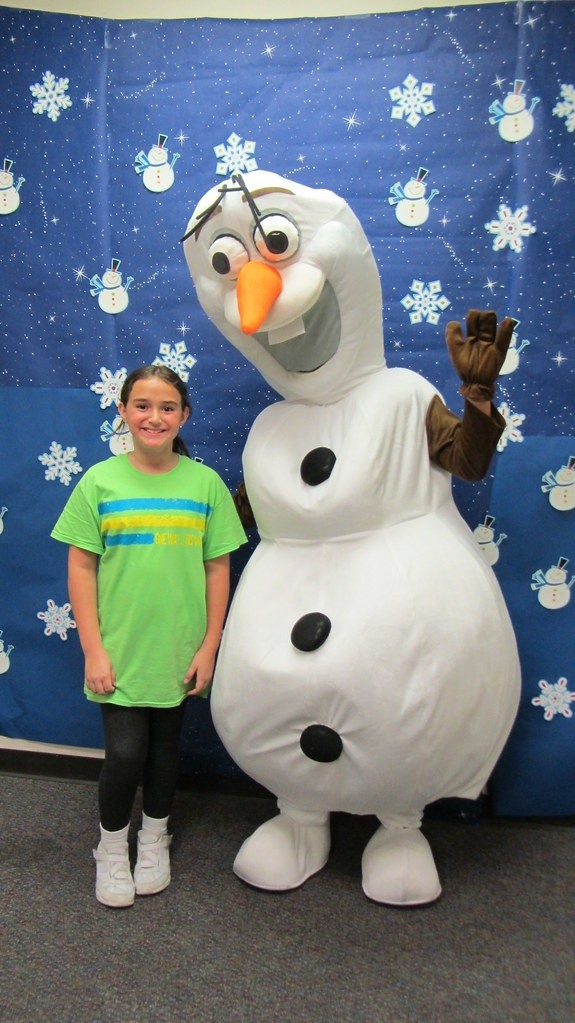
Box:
[92,840,135,907]
[133,830,173,895]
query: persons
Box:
[50,366,248,907]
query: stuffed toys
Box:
[182,168,522,906]
